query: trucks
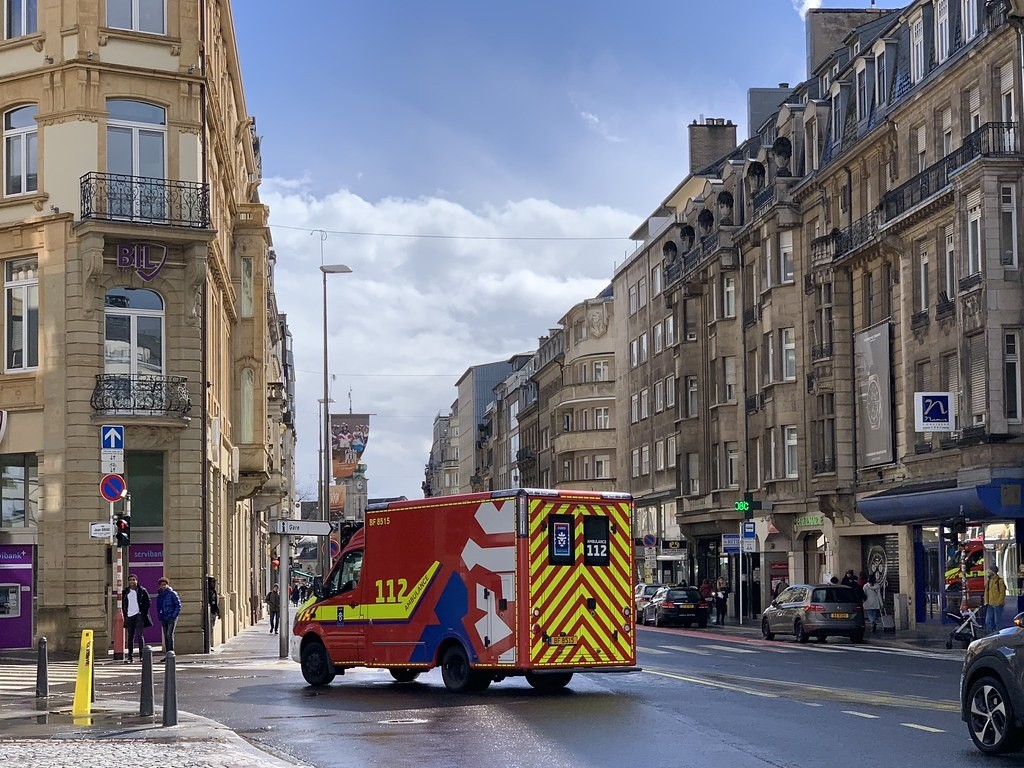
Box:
[290,487,643,694]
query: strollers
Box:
[946,605,982,649]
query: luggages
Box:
[880,607,895,633]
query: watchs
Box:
[867,374,884,430]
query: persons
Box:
[288,583,316,607]
[208,577,220,654]
[265,584,280,634]
[641,578,646,583]
[700,579,714,619]
[122,574,153,664]
[776,577,790,597]
[332,422,368,463]
[676,579,688,588]
[959,606,979,626]
[829,570,868,598]
[863,574,884,633]
[713,576,730,626]
[984,566,1006,636]
[156,577,181,662]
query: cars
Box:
[959,612,1024,755]
[642,585,709,629]
[762,583,865,644]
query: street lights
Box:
[321,264,353,583]
[317,397,335,573]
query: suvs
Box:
[634,583,666,622]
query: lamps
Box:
[232,447,239,482]
[211,416,220,462]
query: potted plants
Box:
[680,223,694,248]
[718,190,734,217]
[771,135,791,166]
[698,209,714,234]
[747,161,764,192]
[663,240,677,261]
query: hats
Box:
[989,565,998,573]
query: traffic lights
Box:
[116,516,130,546]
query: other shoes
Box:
[160,657,166,663]
[270,627,273,633]
[275,631,278,634]
[124,656,133,664]
[139,656,143,662]
